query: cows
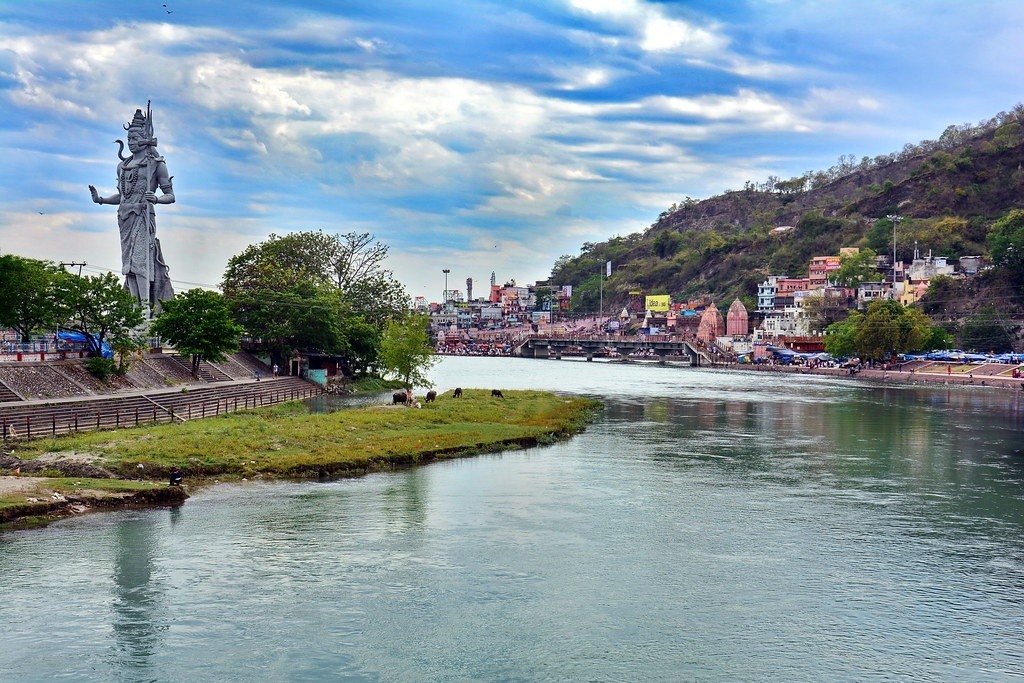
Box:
[491,389,503,398]
[424,391,437,403]
[453,388,462,398]
[393,392,407,405]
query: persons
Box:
[137,461,145,481]
[405,387,413,406]
[9,424,16,436]
[434,339,518,355]
[273,363,278,377]
[88,109,175,319]
[170,467,182,486]
[692,336,1024,379]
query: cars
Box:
[934,350,965,360]
[998,354,1024,362]
[754,357,770,364]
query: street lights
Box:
[597,258,606,324]
[548,277,554,338]
[442,269,450,334]
[887,215,904,300]
